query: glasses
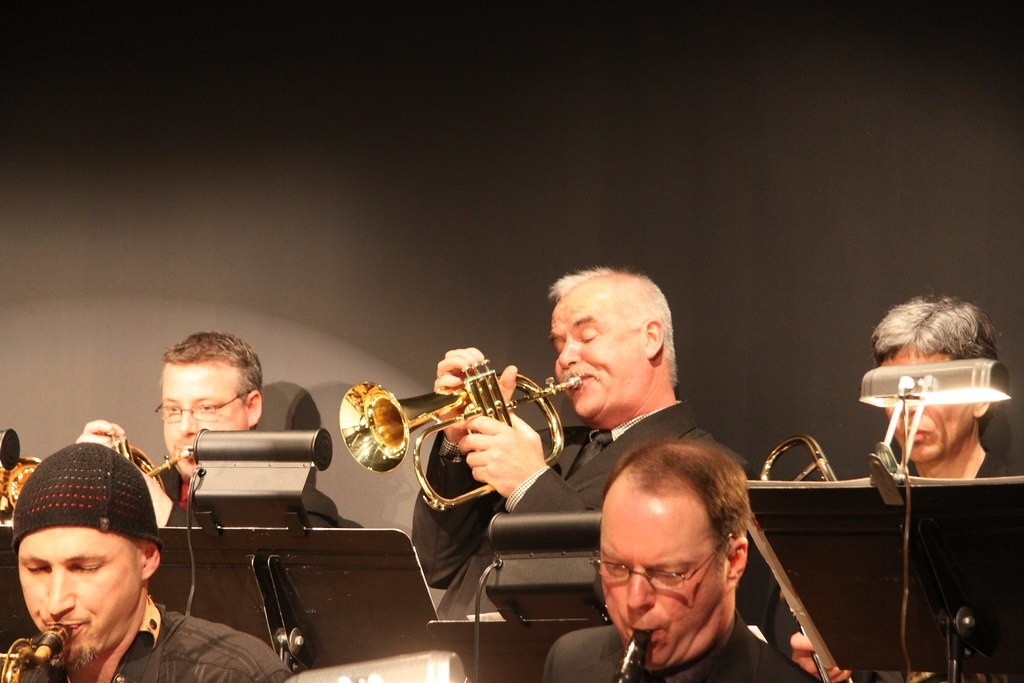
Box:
[589,533,734,588]
[153,394,249,419]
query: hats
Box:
[9,443,165,554]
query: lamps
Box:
[861,358,1011,495]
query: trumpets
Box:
[3,426,195,525]
[337,355,584,511]
[760,432,942,682]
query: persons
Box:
[788,295,1024,683]
[539,436,824,683]
[74,329,340,530]
[411,266,760,622]
[0,441,294,682]
